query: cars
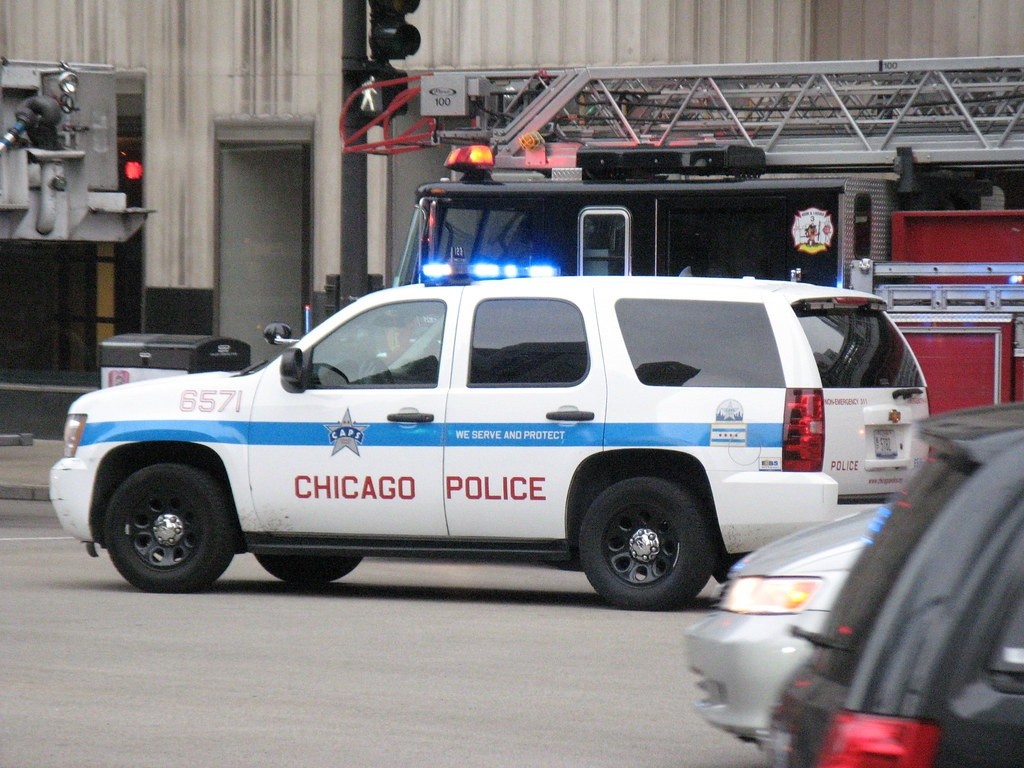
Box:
[763,403,1024,768]
[683,508,907,735]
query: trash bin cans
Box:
[97,333,251,390]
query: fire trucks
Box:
[339,56,1024,418]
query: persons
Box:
[354,310,425,384]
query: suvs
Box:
[45,258,932,616]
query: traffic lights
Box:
[369,0,422,120]
